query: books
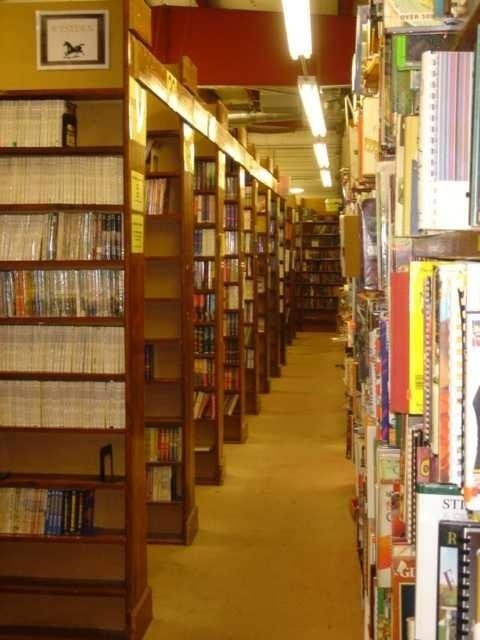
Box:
[0,484,95,537]
[243,180,294,368]
[194,159,219,421]
[2,210,124,318]
[292,208,339,310]
[1,324,125,430]
[1,101,125,205]
[222,173,243,420]
[142,138,167,215]
[338,0,480,640]
[146,425,182,501]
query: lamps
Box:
[281,0,333,188]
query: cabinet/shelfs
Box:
[272,196,301,377]
[226,166,246,445]
[302,219,338,331]
[146,111,194,541]
[340,0,479,639]
[194,150,226,487]
[1,71,148,636]
[247,180,261,417]
[259,189,272,397]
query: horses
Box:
[62,40,85,57]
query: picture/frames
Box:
[35,9,109,70]
